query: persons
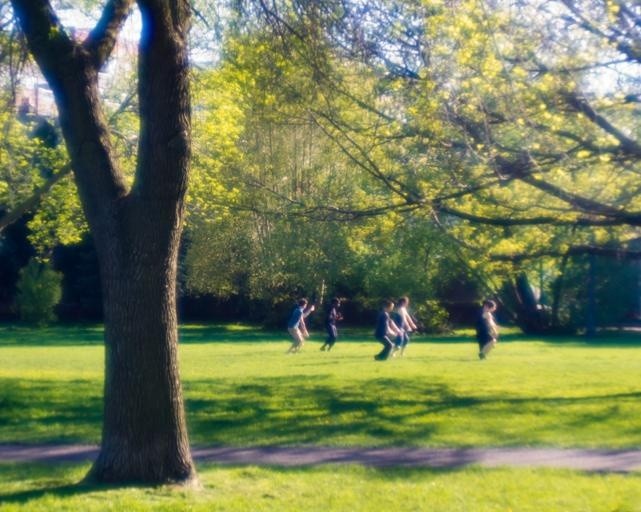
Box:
[476,299,500,362]
[479,302,498,353]
[374,300,404,360]
[286,298,316,354]
[320,298,345,350]
[392,296,418,354]
